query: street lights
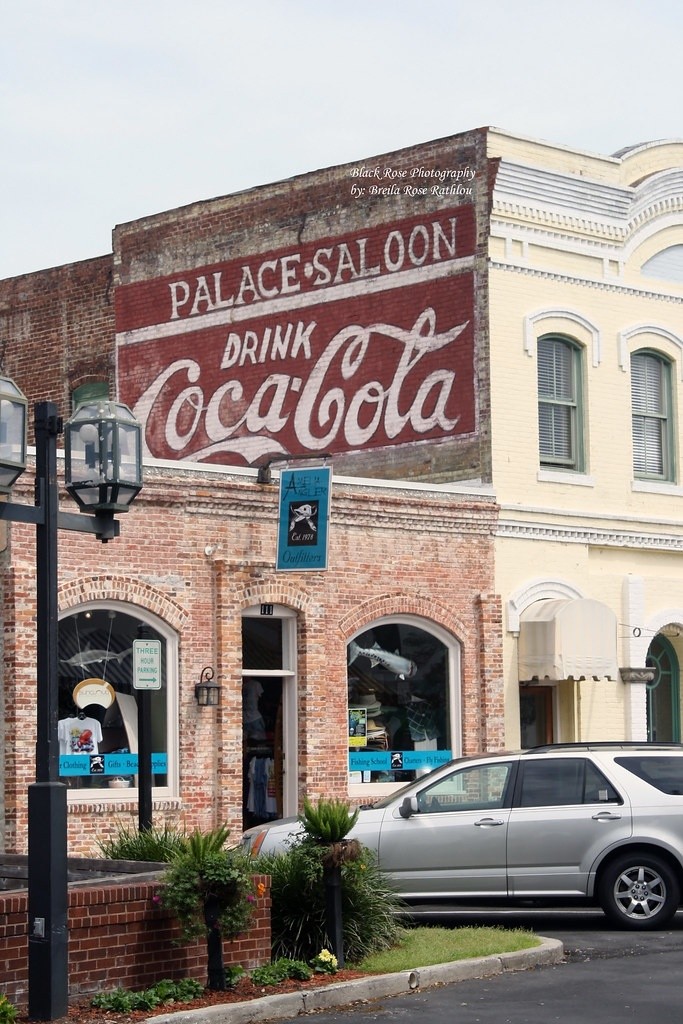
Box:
[0,377,143,1022]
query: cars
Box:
[244,742,682,930]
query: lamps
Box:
[192,667,222,706]
[620,667,657,683]
